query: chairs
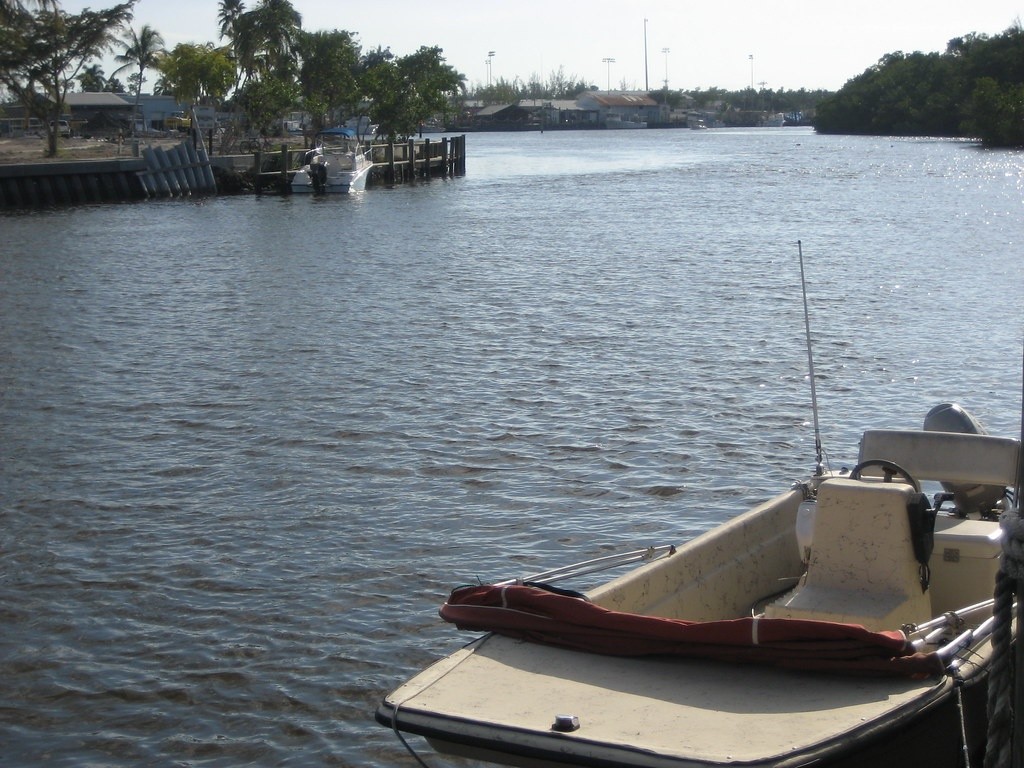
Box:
[764,477,930,648]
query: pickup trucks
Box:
[161,111,192,132]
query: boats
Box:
[373,240,1024,768]
[290,127,375,194]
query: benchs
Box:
[858,430,1020,559]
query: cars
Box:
[48,120,72,139]
